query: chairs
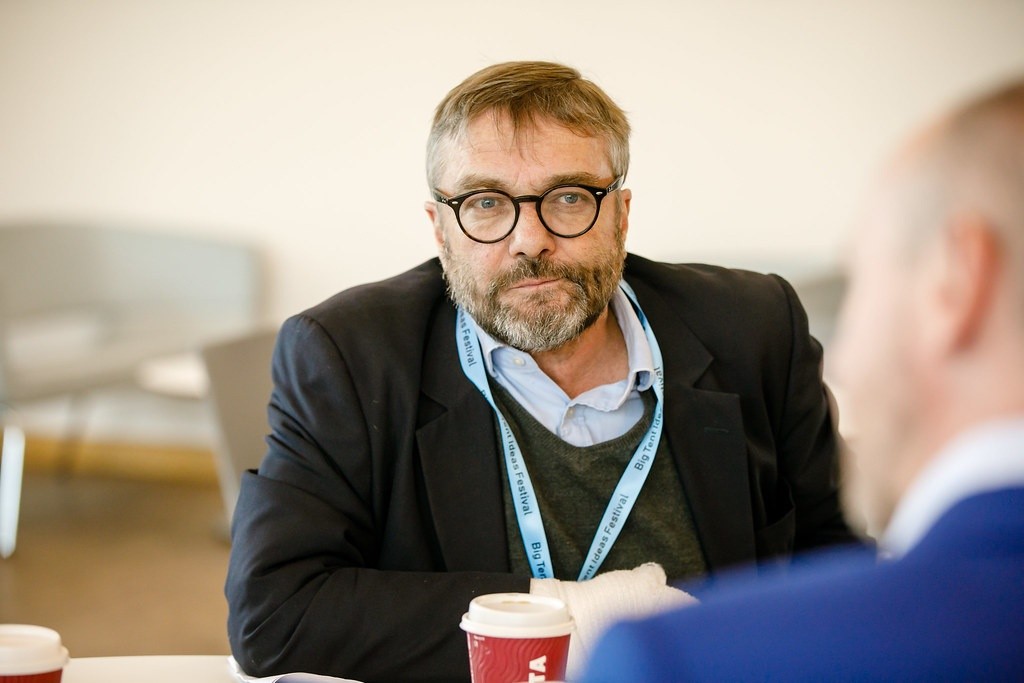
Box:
[0,225,281,561]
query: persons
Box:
[224,61,865,683]
[572,77,1024,683]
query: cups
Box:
[0,623,70,683]
[459,592,572,683]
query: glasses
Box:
[433,175,624,244]
